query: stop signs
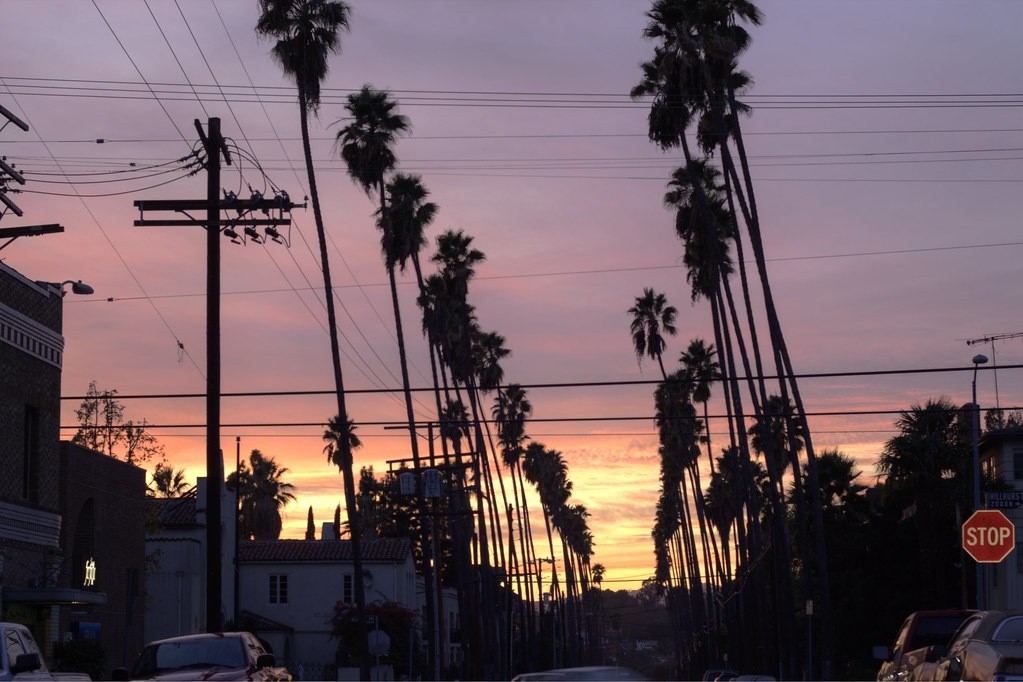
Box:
[961,510,1017,563]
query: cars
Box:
[704,670,776,682]
[113,632,294,682]
[924,609,1022,681]
[512,667,655,680]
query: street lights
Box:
[969,354,990,612]
[538,557,555,578]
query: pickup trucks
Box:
[872,609,984,681]
[0,622,94,681]
